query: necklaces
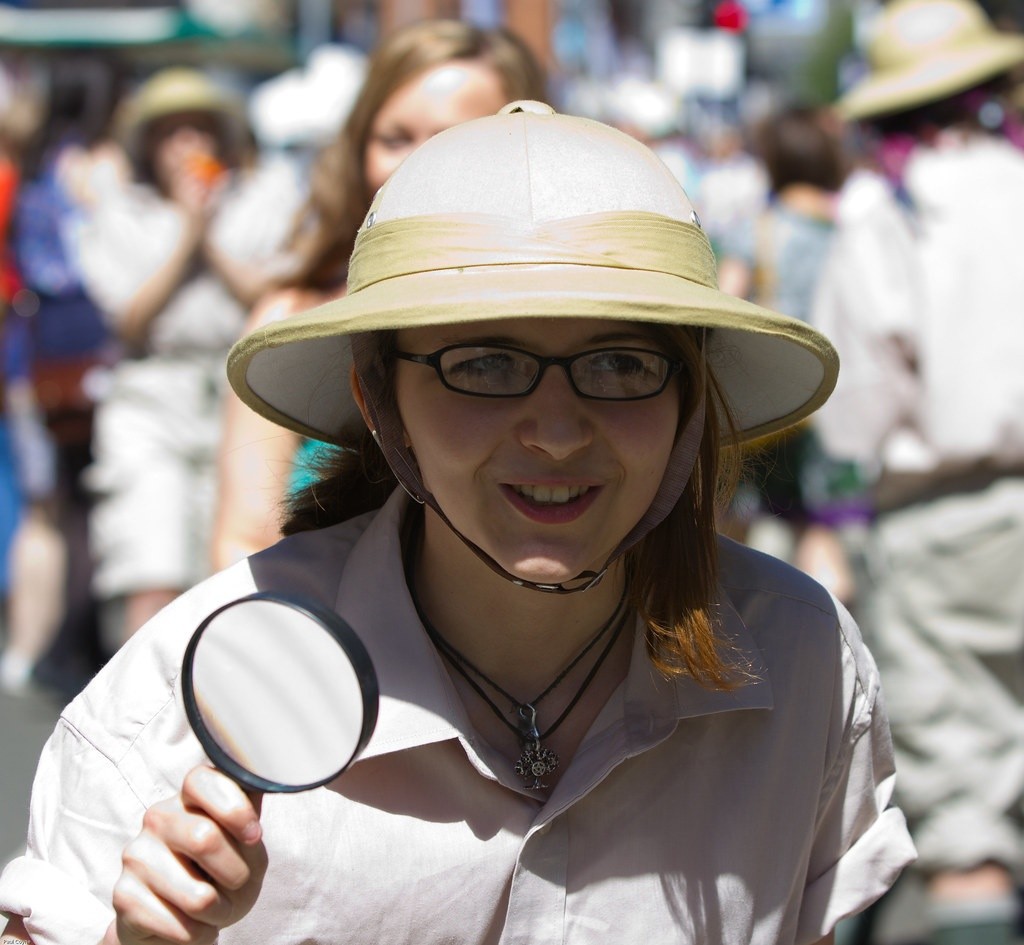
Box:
[415,581,632,791]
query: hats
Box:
[122,71,247,186]
[226,105,836,448]
[829,0,1024,122]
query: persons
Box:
[0,42,365,695]
[209,19,542,578]
[0,100,920,945]
[605,0,1024,945]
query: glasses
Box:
[383,340,689,401]
